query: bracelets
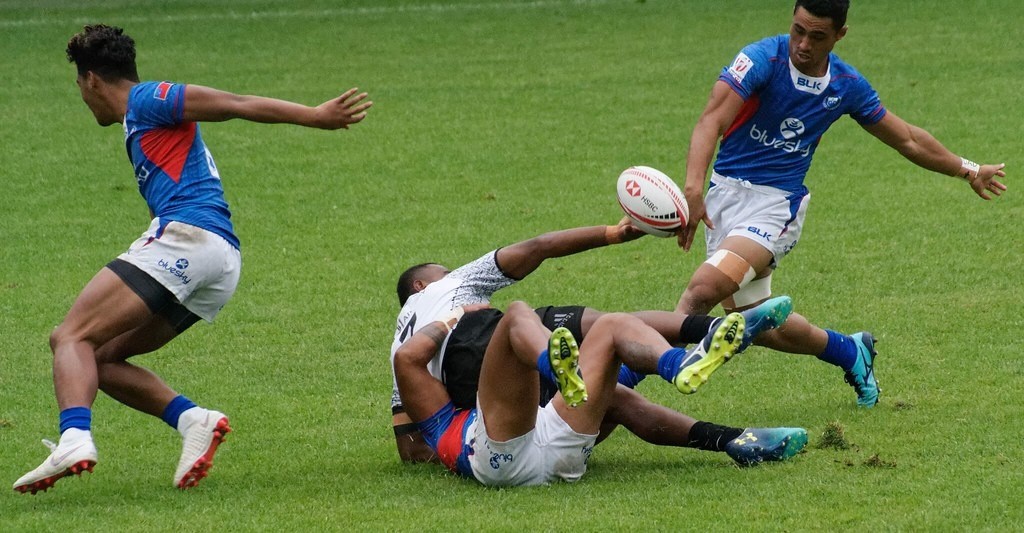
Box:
[953,156,980,183]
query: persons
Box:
[616,0,1006,408]
[391,213,808,489]
[13,24,374,495]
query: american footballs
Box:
[615,164,691,239]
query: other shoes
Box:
[548,327,588,410]
[735,296,793,354]
[672,312,745,394]
[725,428,808,465]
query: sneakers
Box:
[843,332,882,407]
[174,408,232,488]
[13,428,96,495]
